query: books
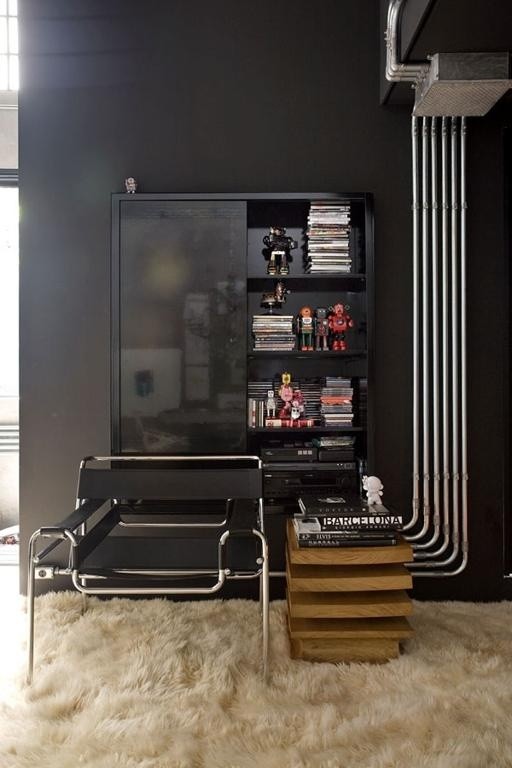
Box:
[292,496,403,548]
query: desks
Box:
[282,514,419,665]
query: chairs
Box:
[24,450,271,683]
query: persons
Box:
[297,303,353,351]
[263,225,295,275]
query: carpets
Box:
[3,588,512,768]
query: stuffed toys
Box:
[264,372,306,419]
[363,476,384,505]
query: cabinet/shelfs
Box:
[108,190,376,514]
